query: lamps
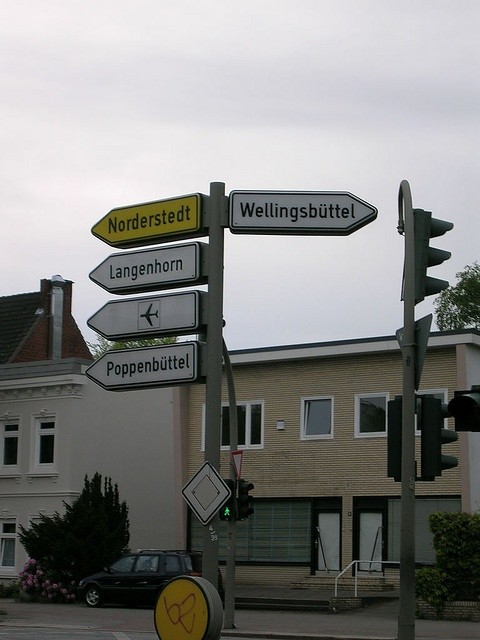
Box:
[276,419,285,431]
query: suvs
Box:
[76,548,226,610]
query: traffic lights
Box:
[384,394,403,484]
[414,207,451,308]
[446,384,478,433]
[219,480,236,521]
[412,394,461,482]
[239,477,256,520]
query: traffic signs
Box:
[227,190,380,238]
[86,191,204,249]
[85,340,205,394]
[82,241,204,292]
[85,288,202,340]
